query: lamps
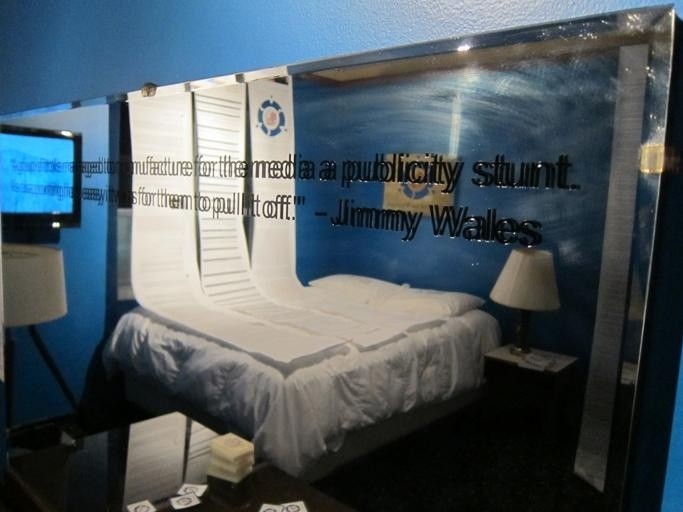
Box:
[488,248,563,357]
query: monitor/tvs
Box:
[0,123,84,245]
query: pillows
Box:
[375,284,487,319]
[307,273,405,308]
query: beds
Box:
[108,274,503,501]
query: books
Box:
[518,348,556,372]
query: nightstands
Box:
[482,341,578,488]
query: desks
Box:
[0,383,348,512]
